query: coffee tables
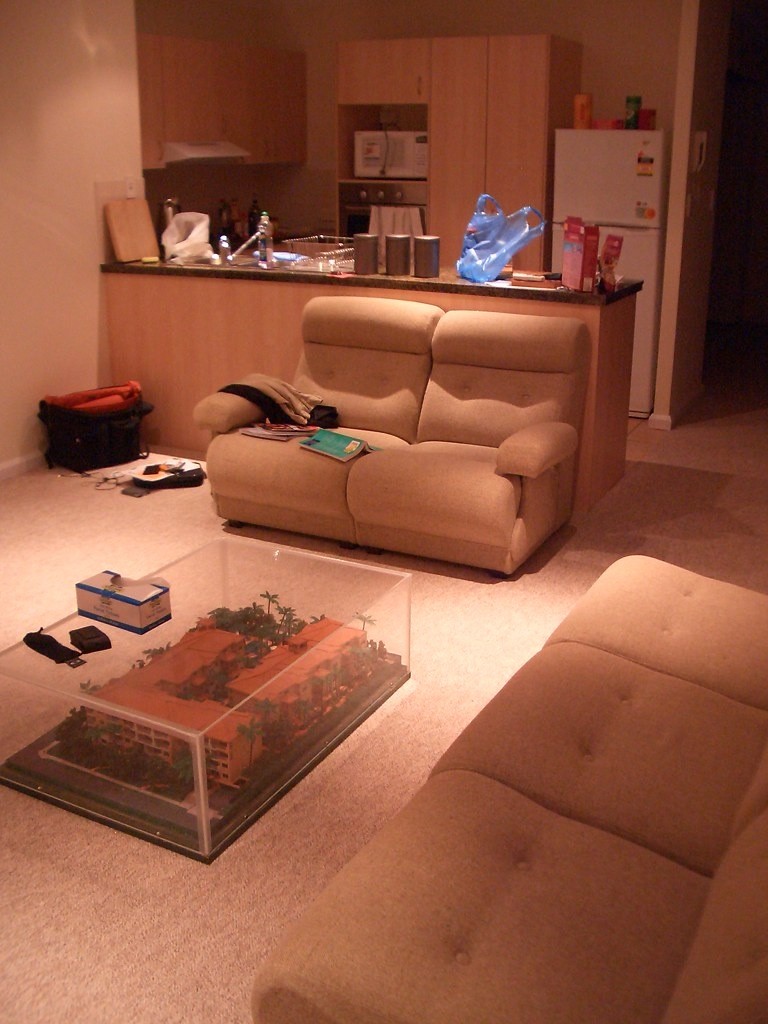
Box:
[0,539,413,864]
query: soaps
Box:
[142,256,160,264]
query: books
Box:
[241,417,322,442]
[297,429,382,462]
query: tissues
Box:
[75,569,174,636]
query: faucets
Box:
[218,230,264,265]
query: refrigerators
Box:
[552,127,670,420]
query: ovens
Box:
[352,131,430,180]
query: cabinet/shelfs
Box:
[136,32,307,168]
[427,34,584,274]
[337,38,431,106]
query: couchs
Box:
[250,555,767,1024]
[193,295,589,580]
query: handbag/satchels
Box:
[456,193,544,283]
[161,211,214,264]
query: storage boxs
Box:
[563,215,600,292]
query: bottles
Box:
[218,235,232,267]
[218,192,262,242]
[257,211,275,269]
